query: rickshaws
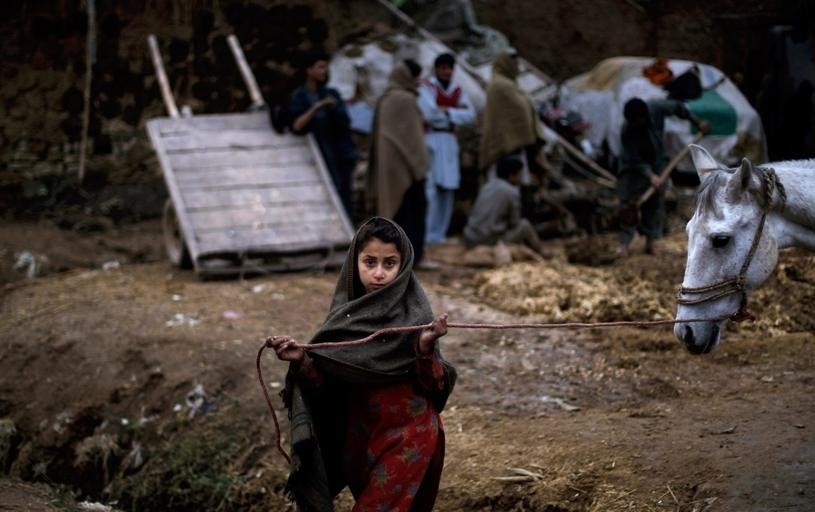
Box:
[142,31,357,285]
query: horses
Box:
[674,144,815,355]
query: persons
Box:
[615,99,710,256]
[477,50,539,223]
[417,51,476,246]
[364,57,435,270]
[288,48,361,216]
[462,157,555,259]
[271,216,457,512]
